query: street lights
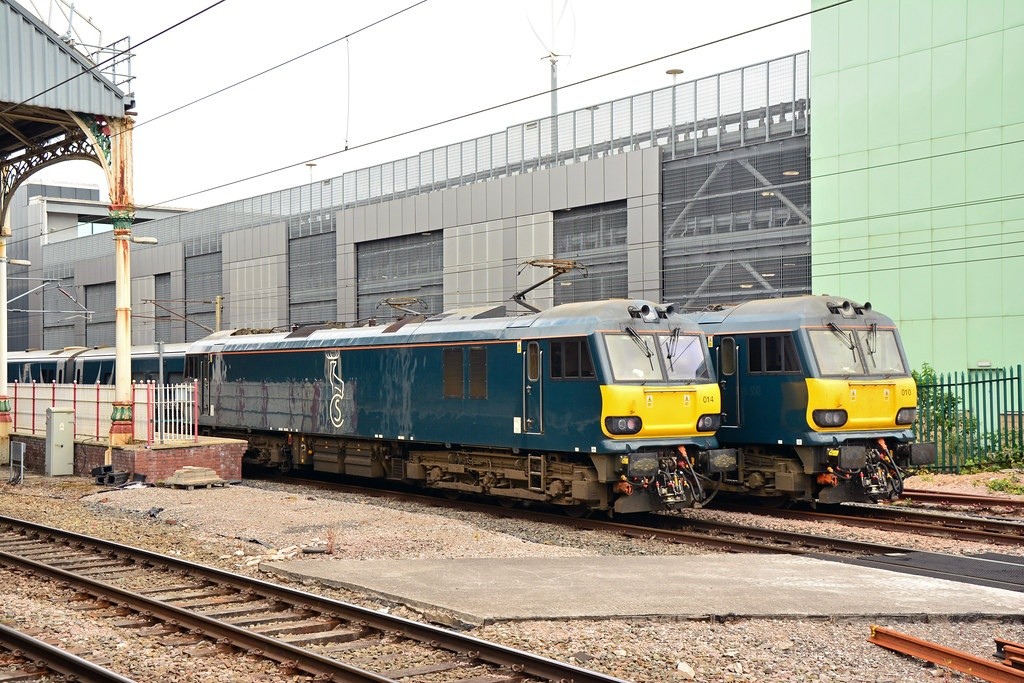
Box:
[666,69,684,158]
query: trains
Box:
[685,294,938,502]
[8,297,735,516]
